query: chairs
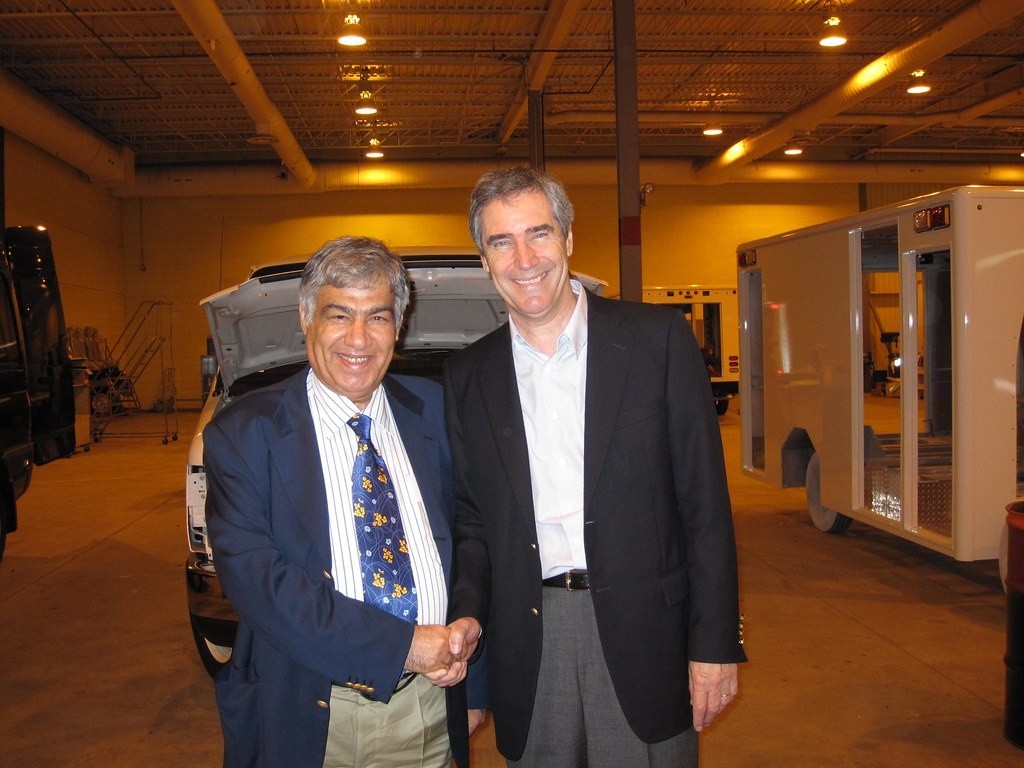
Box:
[68,326,119,381]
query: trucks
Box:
[605,284,740,416]
[734,183,1024,591]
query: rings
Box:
[721,694,731,698]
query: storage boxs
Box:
[886,382,900,396]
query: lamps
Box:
[703,101,722,135]
[784,142,802,155]
[640,183,655,194]
[366,129,384,157]
[907,69,931,94]
[819,0,847,47]
[355,80,377,115]
[336,9,367,46]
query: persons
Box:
[700,345,720,377]
[421,165,749,768]
[203,235,491,768]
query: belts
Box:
[542,571,590,591]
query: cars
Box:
[184,237,606,692]
[0,223,77,573]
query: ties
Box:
[347,413,418,680]
